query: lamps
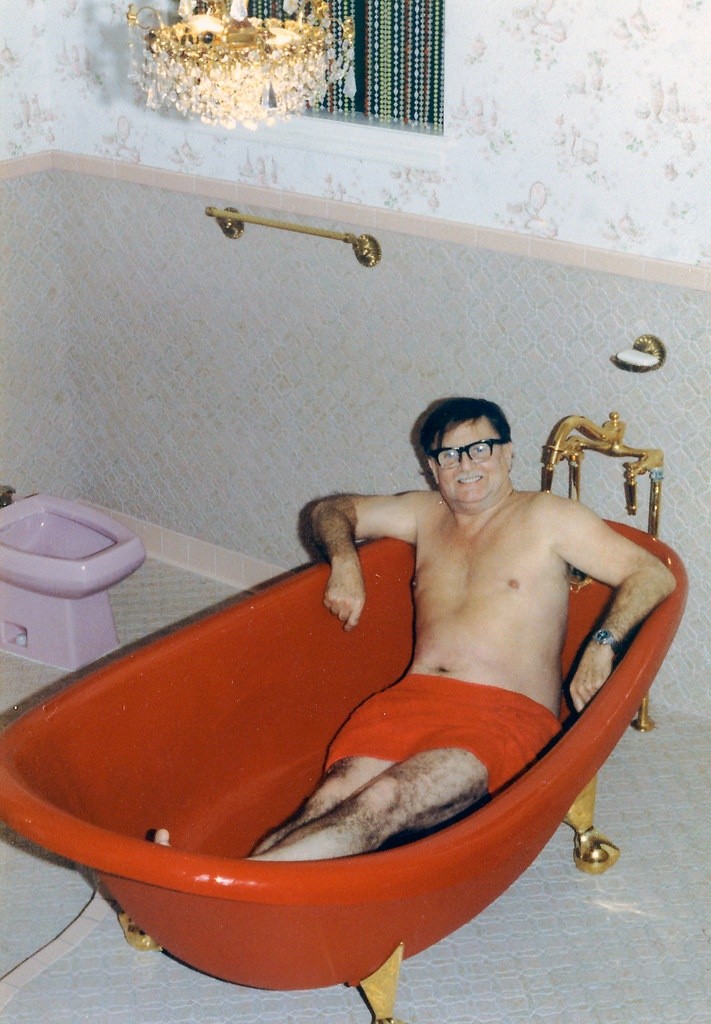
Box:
[125,1,357,132]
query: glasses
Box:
[428,437,507,468]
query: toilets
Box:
[3,493,148,673]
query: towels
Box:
[324,673,562,793]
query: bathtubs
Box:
[0,519,689,992]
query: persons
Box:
[153,398,676,862]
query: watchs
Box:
[593,629,620,654]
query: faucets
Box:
[539,412,624,472]
